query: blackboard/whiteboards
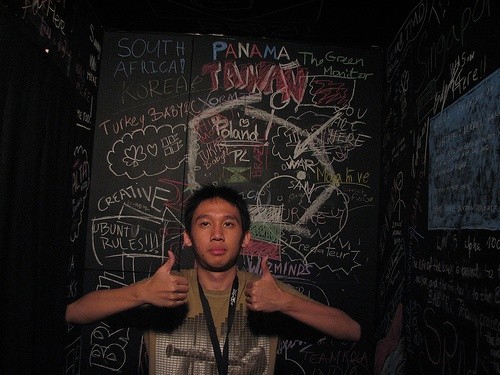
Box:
[1,1,76,73]
[78,32,386,375]
[427,68,500,232]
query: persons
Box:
[66,184,361,375]
